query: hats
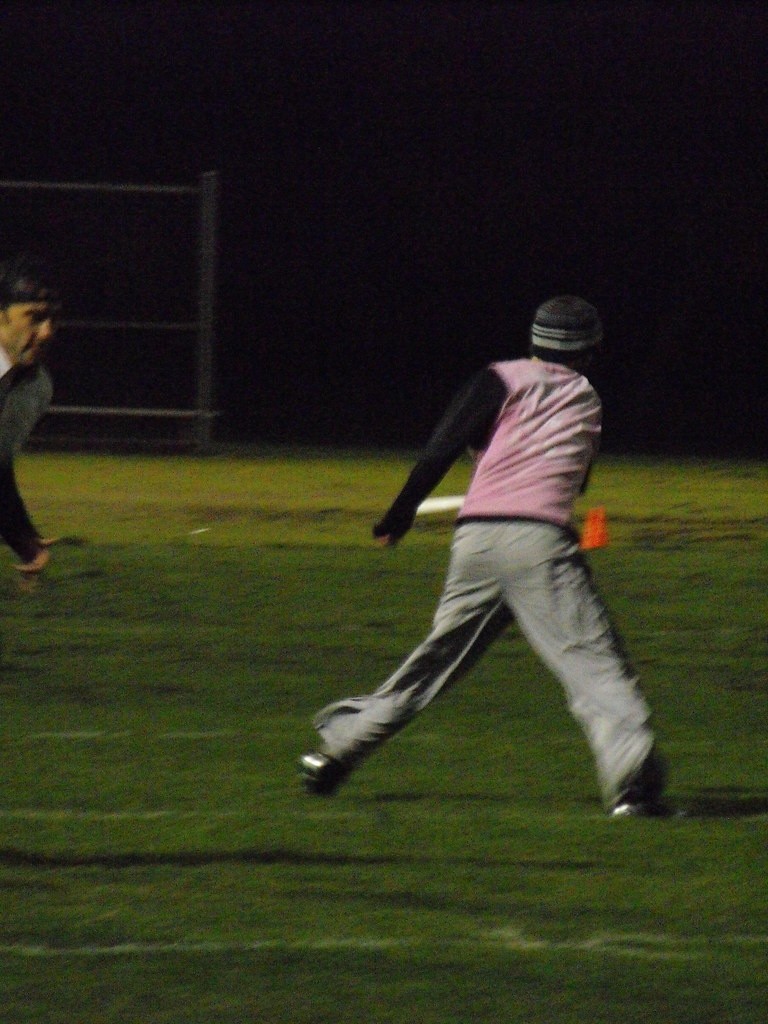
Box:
[0,247,65,302]
[531,296,602,351]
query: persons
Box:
[0,260,63,585]
[296,295,663,816]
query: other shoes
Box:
[298,752,347,795]
[611,787,655,817]
